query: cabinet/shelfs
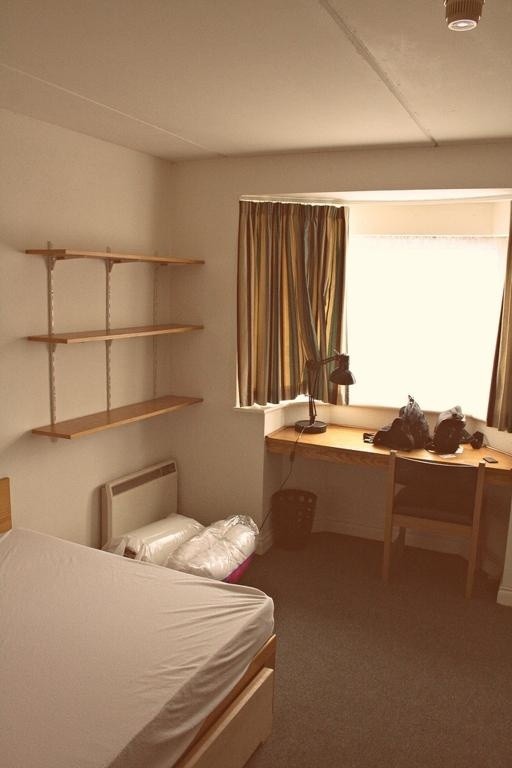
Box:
[26,240,206,440]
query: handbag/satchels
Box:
[432,406,466,455]
[373,395,430,451]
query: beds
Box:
[1,477,277,767]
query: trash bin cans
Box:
[275,489,317,550]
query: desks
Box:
[266,423,512,588]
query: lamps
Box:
[443,0,485,33]
[294,354,356,434]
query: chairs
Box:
[381,450,485,599]
